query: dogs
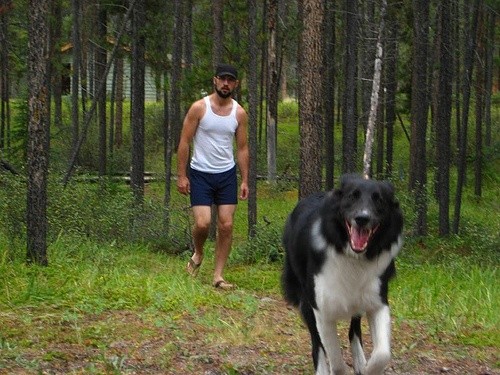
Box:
[279,174,407,375]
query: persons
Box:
[174,64,250,290]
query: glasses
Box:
[216,76,237,83]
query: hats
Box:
[216,64,238,77]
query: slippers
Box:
[212,280,231,291]
[188,258,202,277]
